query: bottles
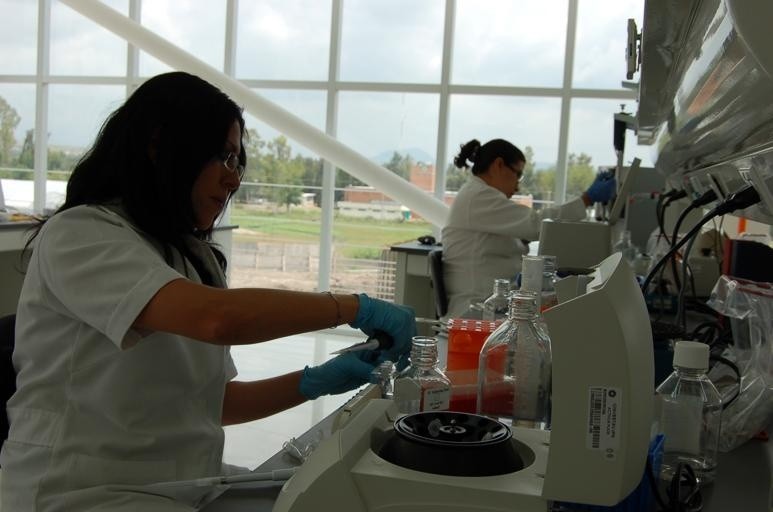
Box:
[389,336,453,417]
[473,287,552,429]
[483,278,511,322]
[613,229,634,261]
[651,334,726,482]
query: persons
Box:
[436,138,619,320]
[0,70,419,511]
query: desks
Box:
[390,235,441,333]
[0,211,241,319]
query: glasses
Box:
[501,160,525,183]
[206,142,248,181]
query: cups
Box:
[446,317,506,414]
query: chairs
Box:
[0,311,18,449]
[428,251,446,321]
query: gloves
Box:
[298,345,384,395]
[585,172,616,206]
[351,294,417,372]
